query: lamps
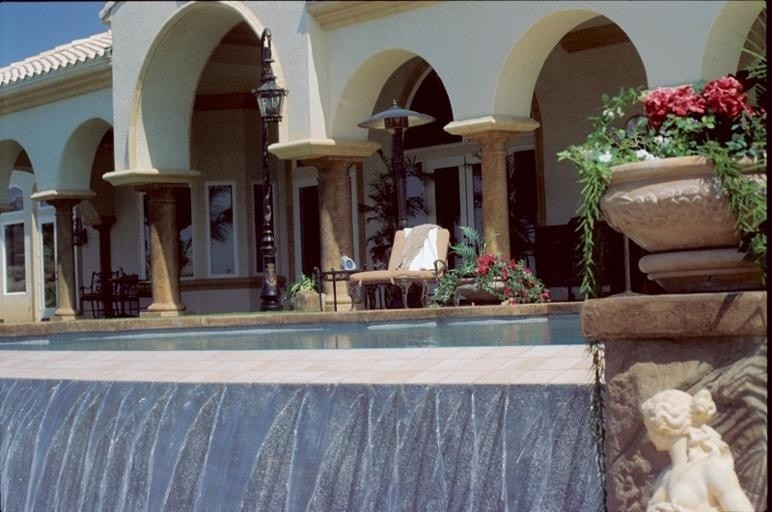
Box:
[73,216,89,247]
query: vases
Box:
[454,277,507,306]
[600,155,766,292]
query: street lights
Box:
[253,21,291,311]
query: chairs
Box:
[348,222,451,309]
[80,270,120,318]
[103,272,141,316]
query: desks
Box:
[313,264,366,312]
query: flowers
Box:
[424,225,552,307]
[555,74,770,297]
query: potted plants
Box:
[281,271,327,312]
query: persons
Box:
[640,388,754,511]
